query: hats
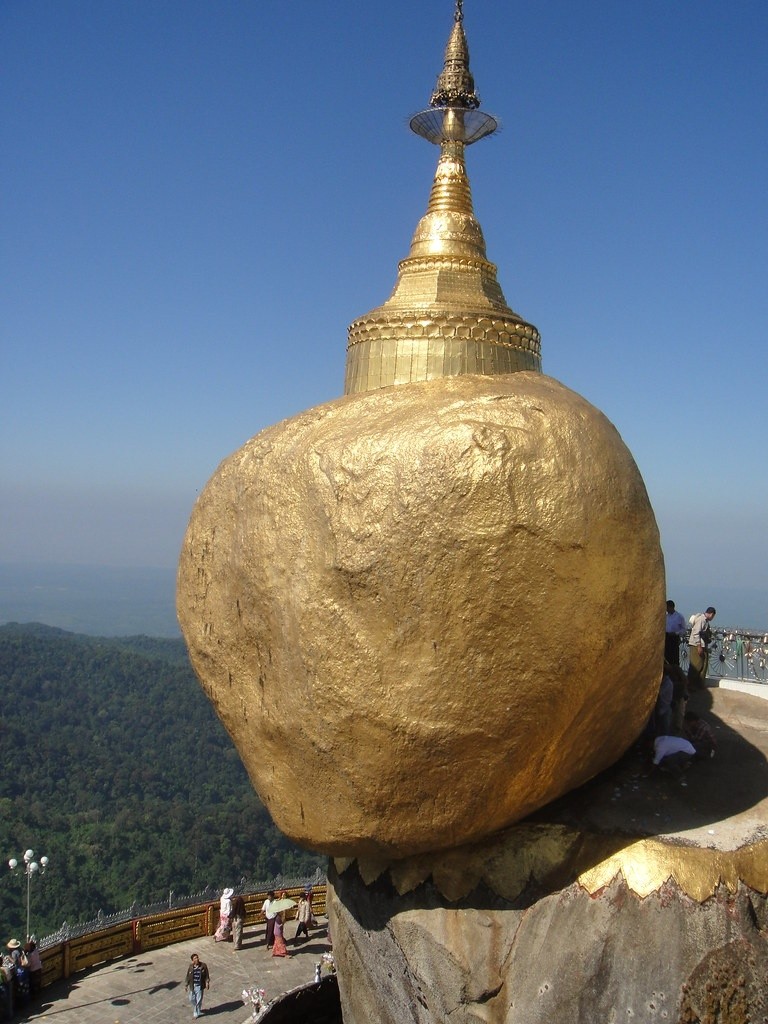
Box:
[305,883,313,891]
[267,891,275,896]
[300,892,306,897]
[689,613,701,628]
[223,888,234,898]
[7,939,21,949]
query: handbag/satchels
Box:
[1,959,15,971]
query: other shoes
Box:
[687,687,696,692]
[212,936,216,942]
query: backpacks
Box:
[19,951,29,967]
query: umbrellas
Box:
[269,898,297,913]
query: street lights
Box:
[8,850,50,944]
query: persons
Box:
[185,954,210,1020]
[641,600,719,776]
[213,883,318,959]
[0,939,42,1024]
